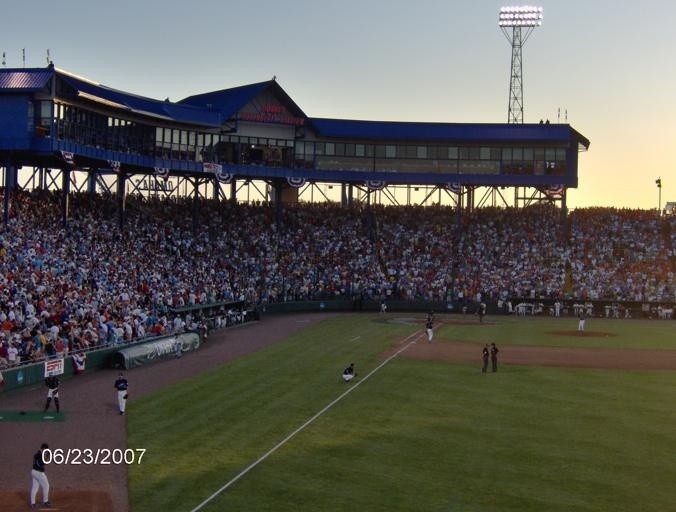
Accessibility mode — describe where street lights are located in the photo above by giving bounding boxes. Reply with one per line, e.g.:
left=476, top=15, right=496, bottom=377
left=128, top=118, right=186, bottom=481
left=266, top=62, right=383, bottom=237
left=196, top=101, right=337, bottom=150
left=497, top=5, right=544, bottom=124
left=656, top=176, right=661, bottom=211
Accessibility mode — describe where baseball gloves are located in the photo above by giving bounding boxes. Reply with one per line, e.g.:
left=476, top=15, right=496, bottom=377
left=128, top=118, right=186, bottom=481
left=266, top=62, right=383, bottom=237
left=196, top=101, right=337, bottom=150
left=53, top=389, right=58, bottom=394
left=123, top=394, right=128, bottom=399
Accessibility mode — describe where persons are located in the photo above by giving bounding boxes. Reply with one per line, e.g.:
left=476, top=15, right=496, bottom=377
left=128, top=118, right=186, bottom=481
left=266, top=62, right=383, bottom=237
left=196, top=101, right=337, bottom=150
left=269, top=200, right=676, bottom=303
left=577, top=311, right=586, bottom=335
left=342, top=364, right=356, bottom=382
left=44, top=372, right=62, bottom=413
left=497, top=301, right=672, bottom=319
left=480, top=301, right=486, bottom=315
left=489, top=343, right=499, bottom=372
left=1, top=181, right=269, bottom=372
left=481, top=344, right=489, bottom=373
left=30, top=443, right=52, bottom=509
left=426, top=319, right=434, bottom=343
left=114, top=372, right=129, bottom=415
left=478, top=305, right=484, bottom=322
left=381, top=303, right=387, bottom=313
